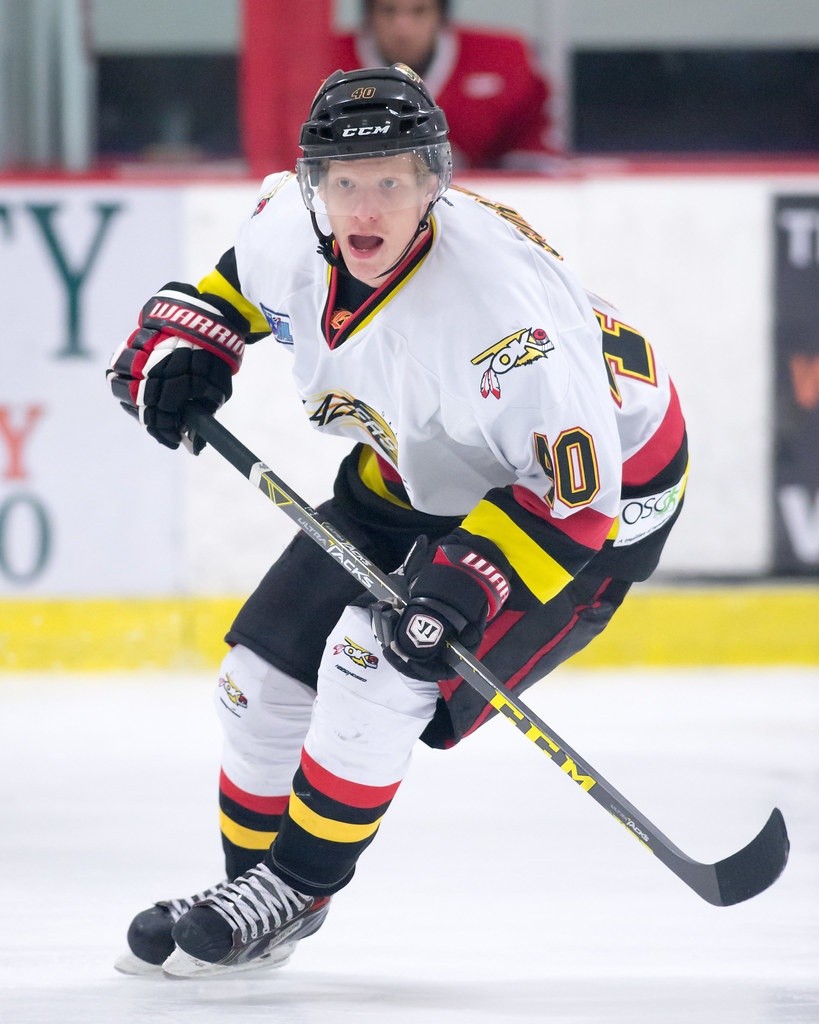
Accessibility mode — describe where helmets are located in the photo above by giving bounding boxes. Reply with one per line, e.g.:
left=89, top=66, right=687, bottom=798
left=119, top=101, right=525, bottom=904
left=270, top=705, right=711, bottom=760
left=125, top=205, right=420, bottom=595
left=294, top=62, right=449, bottom=185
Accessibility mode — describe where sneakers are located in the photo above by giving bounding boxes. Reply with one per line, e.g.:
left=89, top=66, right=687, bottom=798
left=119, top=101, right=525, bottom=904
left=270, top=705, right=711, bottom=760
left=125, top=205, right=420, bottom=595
left=114, top=878, right=231, bottom=975
left=161, top=861, right=332, bottom=978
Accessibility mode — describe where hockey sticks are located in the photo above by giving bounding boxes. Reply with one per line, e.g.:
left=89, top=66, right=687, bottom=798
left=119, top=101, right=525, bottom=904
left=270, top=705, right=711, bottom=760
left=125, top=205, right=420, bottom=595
left=184, top=405, right=791, bottom=908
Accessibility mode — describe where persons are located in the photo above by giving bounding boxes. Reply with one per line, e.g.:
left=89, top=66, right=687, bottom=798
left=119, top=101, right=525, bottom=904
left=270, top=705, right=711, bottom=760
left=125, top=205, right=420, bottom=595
left=331, top=1, right=558, bottom=171
left=111, top=63, right=688, bottom=981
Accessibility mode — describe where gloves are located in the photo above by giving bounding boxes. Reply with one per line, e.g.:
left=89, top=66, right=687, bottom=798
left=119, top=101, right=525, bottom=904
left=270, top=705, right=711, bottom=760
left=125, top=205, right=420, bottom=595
left=370, top=527, right=514, bottom=682
left=104, top=281, right=251, bottom=450
